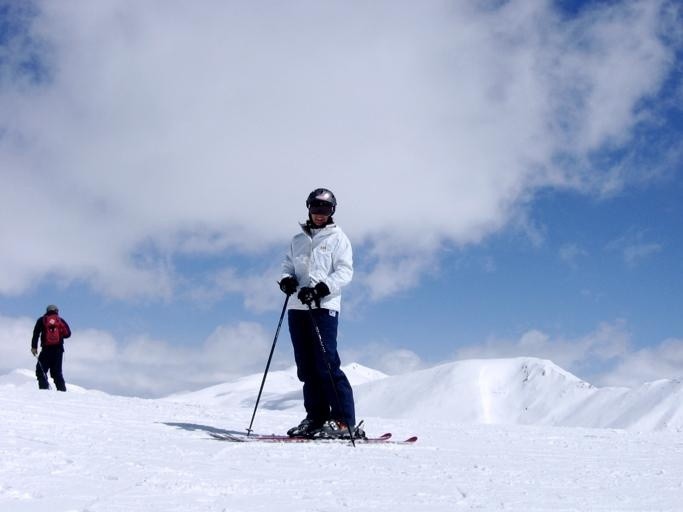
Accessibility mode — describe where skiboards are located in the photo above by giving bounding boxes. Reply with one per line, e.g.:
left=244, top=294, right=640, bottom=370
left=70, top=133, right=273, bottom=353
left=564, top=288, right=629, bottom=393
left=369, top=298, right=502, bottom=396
left=207, top=432, right=418, bottom=444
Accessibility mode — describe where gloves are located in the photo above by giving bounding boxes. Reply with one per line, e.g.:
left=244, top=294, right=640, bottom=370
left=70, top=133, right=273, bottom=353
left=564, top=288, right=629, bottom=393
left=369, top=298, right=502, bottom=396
left=280, top=277, right=297, bottom=295
left=298, top=284, right=324, bottom=304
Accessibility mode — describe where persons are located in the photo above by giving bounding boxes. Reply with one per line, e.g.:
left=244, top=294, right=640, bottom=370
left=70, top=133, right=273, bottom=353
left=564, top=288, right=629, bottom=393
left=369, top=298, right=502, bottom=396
left=277, top=188, right=365, bottom=439
left=30, top=303, right=72, bottom=393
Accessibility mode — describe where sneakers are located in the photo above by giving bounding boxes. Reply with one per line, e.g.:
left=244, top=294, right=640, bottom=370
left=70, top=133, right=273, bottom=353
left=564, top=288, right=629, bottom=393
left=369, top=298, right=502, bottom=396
left=288, top=417, right=321, bottom=437
left=313, top=421, right=366, bottom=439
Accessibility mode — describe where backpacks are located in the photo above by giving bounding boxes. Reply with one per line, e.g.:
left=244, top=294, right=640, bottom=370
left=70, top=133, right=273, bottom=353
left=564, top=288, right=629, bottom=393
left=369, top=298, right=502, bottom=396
left=43, top=316, right=63, bottom=346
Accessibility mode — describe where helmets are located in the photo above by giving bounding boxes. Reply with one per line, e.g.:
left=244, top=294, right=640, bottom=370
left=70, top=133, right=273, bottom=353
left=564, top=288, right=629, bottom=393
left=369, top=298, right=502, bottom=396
left=307, top=188, right=337, bottom=208
left=46, top=304, right=58, bottom=312
